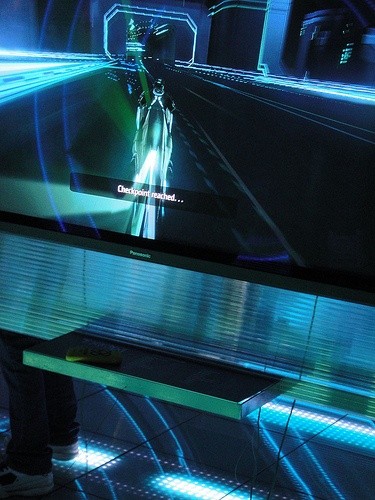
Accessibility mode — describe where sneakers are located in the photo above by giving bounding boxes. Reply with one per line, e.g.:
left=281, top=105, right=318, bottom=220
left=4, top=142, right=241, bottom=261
left=0, top=465, right=54, bottom=498
left=48, top=440, right=79, bottom=461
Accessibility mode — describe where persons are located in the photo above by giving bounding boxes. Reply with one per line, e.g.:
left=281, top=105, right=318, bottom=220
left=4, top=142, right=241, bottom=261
left=0, top=329, right=81, bottom=499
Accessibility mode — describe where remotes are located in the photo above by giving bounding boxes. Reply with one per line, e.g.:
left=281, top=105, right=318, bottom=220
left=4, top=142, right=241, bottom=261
left=65, top=346, right=123, bottom=364
